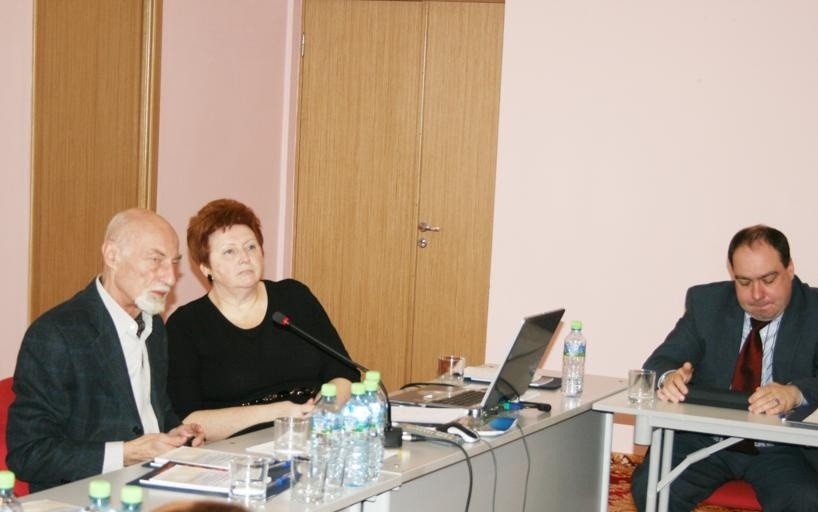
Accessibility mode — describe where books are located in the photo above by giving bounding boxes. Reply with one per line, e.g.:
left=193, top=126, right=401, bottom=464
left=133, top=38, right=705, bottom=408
left=132, top=441, right=281, bottom=496
left=462, top=361, right=545, bottom=382
left=469, top=375, right=555, bottom=387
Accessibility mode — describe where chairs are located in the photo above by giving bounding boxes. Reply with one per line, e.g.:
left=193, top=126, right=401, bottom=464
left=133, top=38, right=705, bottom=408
left=0, top=377, right=28, bottom=496
left=700, top=480, right=761, bottom=512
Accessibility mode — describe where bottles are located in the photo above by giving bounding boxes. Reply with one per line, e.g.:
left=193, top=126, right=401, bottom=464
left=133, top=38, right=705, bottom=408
left=84, top=479, right=117, bottom=512
left=119, top=484, right=145, bottom=511
left=562, top=319, right=587, bottom=396
left=314, top=369, right=388, bottom=493
left=0, top=469, right=23, bottom=512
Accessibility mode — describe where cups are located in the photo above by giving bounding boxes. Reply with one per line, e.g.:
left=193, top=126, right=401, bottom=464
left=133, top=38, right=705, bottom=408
left=626, top=368, right=657, bottom=406
left=290, top=454, right=327, bottom=503
left=274, top=416, right=312, bottom=454
left=437, top=353, right=466, bottom=387
left=228, top=452, right=270, bottom=511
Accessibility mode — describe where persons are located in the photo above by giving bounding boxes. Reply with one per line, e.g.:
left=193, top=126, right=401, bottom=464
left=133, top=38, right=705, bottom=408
left=628, top=224, right=818, bottom=511
left=162, top=198, right=362, bottom=444
left=4, top=207, right=208, bottom=493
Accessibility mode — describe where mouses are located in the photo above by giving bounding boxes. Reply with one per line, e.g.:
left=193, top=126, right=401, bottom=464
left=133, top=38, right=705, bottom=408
left=442, top=422, right=480, bottom=443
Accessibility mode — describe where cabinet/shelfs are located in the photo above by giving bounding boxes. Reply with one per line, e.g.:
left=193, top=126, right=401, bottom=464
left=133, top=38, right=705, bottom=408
left=291, top=0, right=506, bottom=394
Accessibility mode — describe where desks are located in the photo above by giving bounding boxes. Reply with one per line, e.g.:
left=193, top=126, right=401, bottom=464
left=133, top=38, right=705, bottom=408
left=0, top=368, right=630, bottom=512
left=593, top=382, right=818, bottom=512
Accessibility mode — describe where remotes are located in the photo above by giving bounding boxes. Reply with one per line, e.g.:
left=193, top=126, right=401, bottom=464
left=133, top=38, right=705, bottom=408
left=398, top=421, right=463, bottom=444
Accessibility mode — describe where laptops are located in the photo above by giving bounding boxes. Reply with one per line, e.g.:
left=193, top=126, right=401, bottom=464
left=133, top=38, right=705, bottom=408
left=388, top=308, right=565, bottom=420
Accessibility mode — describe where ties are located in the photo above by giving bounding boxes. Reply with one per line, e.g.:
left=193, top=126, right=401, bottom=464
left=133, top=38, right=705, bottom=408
left=724, top=317, right=772, bottom=454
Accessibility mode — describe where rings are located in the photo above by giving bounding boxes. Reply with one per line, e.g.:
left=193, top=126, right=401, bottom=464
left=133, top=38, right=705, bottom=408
left=773, top=397, right=780, bottom=406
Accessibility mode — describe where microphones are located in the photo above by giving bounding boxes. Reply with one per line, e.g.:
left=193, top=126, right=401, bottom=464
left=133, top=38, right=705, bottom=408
left=272, top=311, right=402, bottom=449
